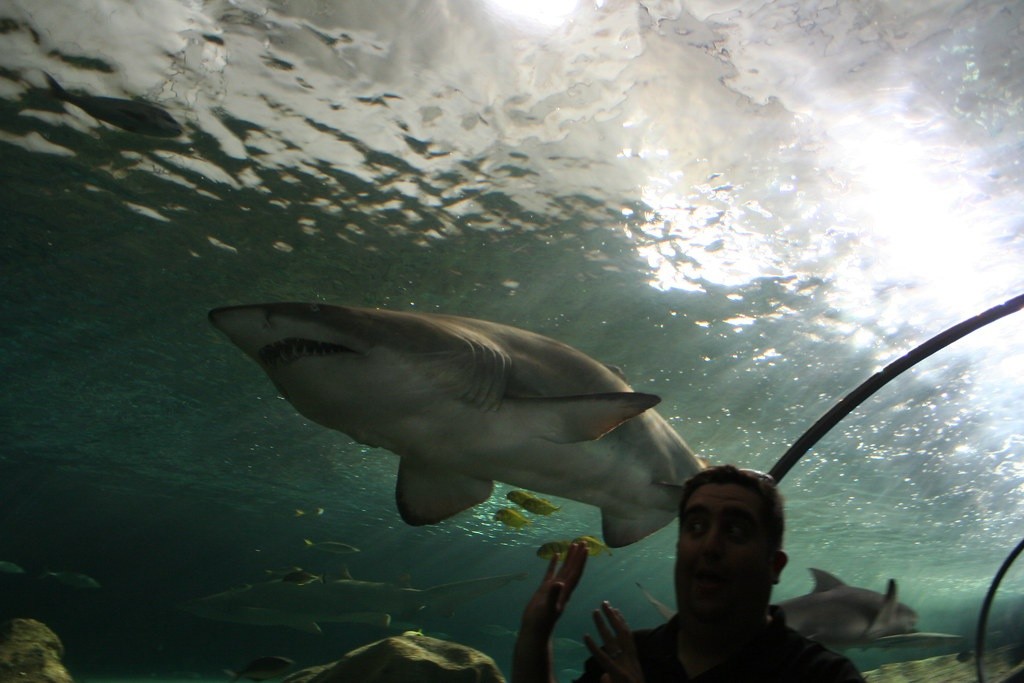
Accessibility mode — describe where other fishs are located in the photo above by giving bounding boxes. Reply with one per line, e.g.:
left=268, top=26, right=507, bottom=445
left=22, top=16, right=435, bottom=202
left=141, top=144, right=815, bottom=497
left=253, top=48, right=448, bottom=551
left=490, top=488, right=562, bottom=529
left=535, top=535, right=613, bottom=564
left=272, top=506, right=430, bottom=639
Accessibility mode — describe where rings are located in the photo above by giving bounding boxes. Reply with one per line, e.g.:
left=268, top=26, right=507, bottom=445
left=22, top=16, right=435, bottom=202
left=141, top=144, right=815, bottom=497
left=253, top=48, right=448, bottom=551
left=611, top=649, right=624, bottom=659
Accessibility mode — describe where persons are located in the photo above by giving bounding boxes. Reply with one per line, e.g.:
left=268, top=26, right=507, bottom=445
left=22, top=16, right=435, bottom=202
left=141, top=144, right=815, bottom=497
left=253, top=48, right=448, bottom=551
left=512, top=466, right=865, bottom=683
